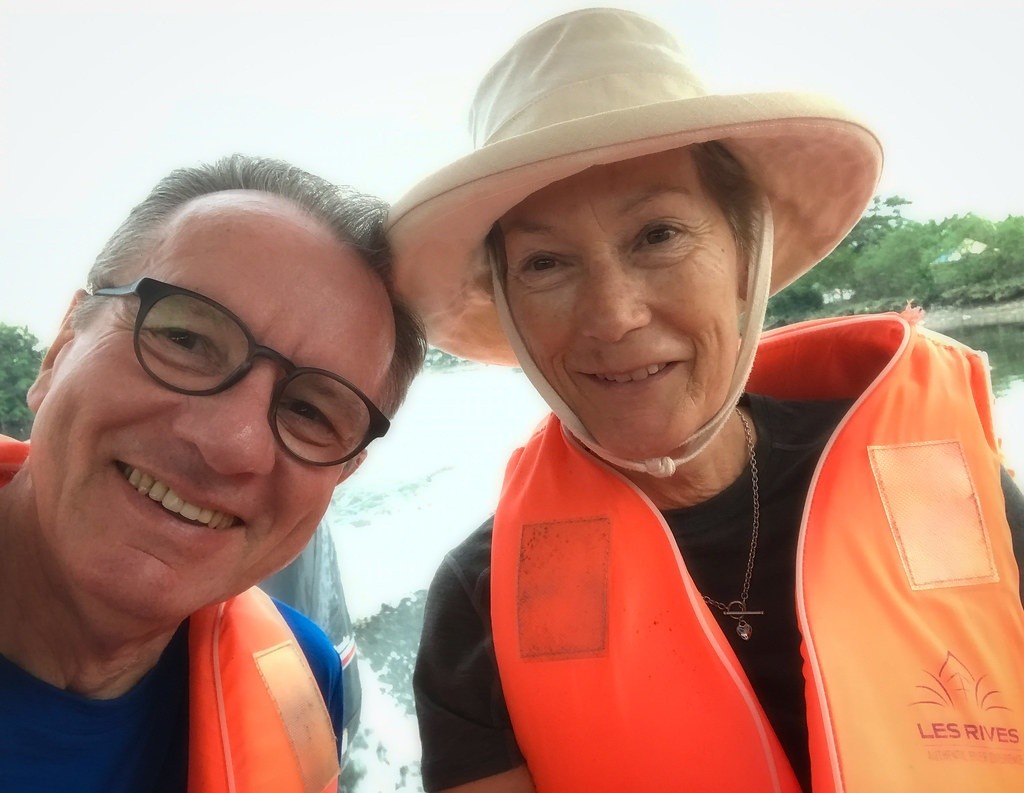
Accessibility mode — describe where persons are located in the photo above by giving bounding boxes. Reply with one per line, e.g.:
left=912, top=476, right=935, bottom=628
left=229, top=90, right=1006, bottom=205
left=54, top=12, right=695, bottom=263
left=0, top=158, right=426, bottom=793
left=387, top=7, right=1024, bottom=793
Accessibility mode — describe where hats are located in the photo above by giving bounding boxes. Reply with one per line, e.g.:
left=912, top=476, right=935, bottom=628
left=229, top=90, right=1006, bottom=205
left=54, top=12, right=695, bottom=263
left=385, top=8, right=885, bottom=366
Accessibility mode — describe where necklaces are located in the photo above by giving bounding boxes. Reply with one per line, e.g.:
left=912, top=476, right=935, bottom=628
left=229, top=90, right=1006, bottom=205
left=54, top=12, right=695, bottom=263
left=702, top=406, right=759, bottom=640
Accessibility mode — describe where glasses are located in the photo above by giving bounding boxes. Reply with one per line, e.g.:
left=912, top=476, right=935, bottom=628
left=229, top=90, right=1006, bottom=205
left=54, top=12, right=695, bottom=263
left=93, top=276, right=392, bottom=468
